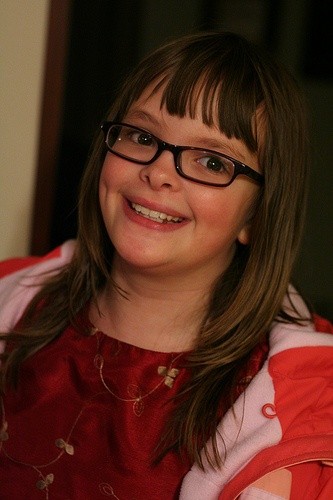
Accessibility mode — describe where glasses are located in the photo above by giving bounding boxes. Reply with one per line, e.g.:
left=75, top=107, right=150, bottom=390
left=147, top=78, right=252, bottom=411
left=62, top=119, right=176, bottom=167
left=99, top=120, right=263, bottom=190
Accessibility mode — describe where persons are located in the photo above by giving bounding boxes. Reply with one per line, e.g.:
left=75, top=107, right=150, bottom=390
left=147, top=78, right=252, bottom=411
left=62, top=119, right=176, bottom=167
left=0, top=29, right=333, bottom=500
